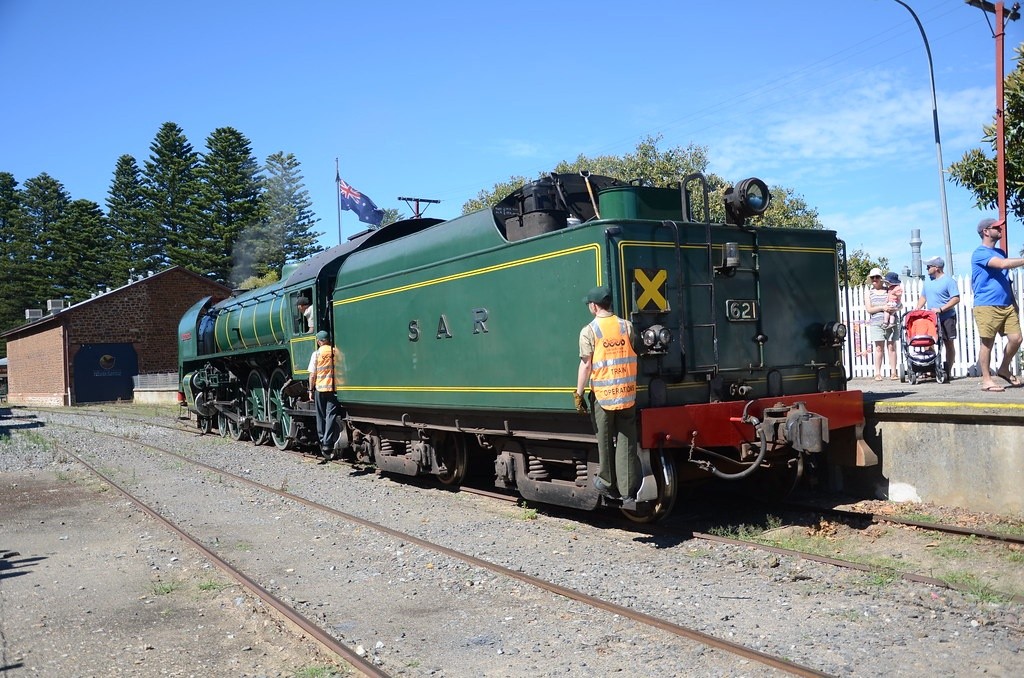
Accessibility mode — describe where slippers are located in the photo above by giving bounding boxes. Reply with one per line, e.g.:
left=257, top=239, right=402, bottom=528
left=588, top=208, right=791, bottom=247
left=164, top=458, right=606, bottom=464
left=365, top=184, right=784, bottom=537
left=997, top=373, right=1021, bottom=386
left=981, top=386, right=1005, bottom=392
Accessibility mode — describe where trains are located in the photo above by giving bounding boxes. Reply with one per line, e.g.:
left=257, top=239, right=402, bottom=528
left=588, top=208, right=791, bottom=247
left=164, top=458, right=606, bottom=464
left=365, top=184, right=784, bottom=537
left=176, top=169, right=878, bottom=528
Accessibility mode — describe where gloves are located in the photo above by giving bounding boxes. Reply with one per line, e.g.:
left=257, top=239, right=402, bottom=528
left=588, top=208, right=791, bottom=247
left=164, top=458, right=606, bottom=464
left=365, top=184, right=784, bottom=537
left=574, top=389, right=588, bottom=415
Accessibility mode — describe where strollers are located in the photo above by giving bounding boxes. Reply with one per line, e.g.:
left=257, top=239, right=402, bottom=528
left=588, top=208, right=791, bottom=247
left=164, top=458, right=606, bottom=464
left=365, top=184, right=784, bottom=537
left=898, top=309, right=949, bottom=385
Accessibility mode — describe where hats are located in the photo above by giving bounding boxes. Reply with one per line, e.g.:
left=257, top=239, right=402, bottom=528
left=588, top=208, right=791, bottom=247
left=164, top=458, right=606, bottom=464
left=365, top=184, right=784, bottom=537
left=294, top=296, right=309, bottom=306
left=977, top=218, right=1005, bottom=234
left=867, top=268, right=882, bottom=277
left=584, top=286, right=610, bottom=305
left=316, top=330, right=328, bottom=340
left=923, top=256, right=944, bottom=267
left=881, top=272, right=901, bottom=284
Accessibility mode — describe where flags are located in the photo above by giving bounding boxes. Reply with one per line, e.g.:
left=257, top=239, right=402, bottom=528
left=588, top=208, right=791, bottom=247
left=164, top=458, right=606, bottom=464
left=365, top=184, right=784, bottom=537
left=340, top=179, right=384, bottom=227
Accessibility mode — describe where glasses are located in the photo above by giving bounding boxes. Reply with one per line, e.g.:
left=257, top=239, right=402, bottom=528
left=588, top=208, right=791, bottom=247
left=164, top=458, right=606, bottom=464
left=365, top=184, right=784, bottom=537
left=870, top=275, right=880, bottom=279
left=927, top=265, right=935, bottom=268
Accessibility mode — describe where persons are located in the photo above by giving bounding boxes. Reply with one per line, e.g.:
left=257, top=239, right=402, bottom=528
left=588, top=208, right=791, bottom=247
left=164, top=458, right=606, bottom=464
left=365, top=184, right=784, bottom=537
left=574, top=286, right=637, bottom=504
left=915, top=256, right=960, bottom=383
left=971, top=218, right=1024, bottom=392
left=880, top=272, right=902, bottom=329
left=865, top=268, right=903, bottom=381
left=307, top=331, right=346, bottom=461
left=294, top=296, right=313, bottom=334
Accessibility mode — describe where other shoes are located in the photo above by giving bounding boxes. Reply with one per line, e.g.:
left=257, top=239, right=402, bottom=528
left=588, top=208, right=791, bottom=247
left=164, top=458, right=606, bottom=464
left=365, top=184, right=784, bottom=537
left=612, top=497, right=635, bottom=505
left=881, top=323, right=896, bottom=330
left=592, top=477, right=614, bottom=492
left=322, top=449, right=332, bottom=460
left=890, top=375, right=897, bottom=380
left=874, top=375, right=882, bottom=381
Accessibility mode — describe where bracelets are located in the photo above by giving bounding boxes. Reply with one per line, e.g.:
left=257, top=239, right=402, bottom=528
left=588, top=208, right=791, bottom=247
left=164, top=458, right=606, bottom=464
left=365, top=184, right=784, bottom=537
left=940, top=308, right=943, bottom=313
left=308, top=390, right=313, bottom=392
